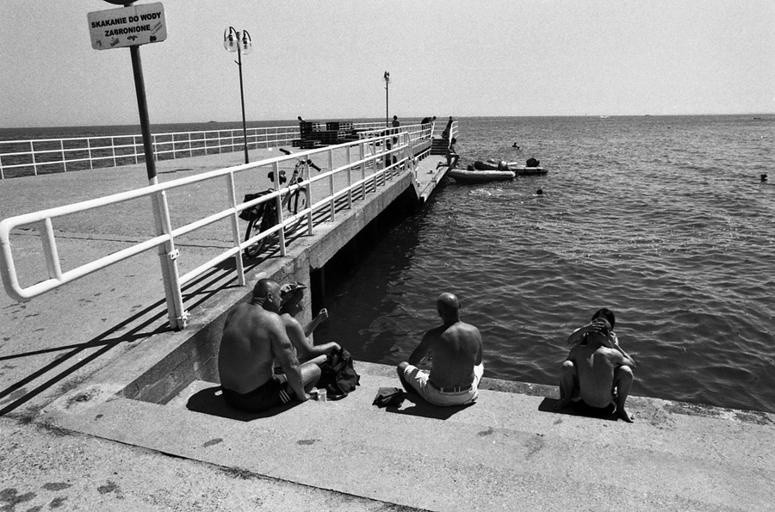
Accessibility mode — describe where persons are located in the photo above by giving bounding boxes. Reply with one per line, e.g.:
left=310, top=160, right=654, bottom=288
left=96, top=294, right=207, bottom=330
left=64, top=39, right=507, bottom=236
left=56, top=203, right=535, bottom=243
left=553, top=307, right=635, bottom=424
left=420, top=117, right=432, bottom=140
left=512, top=142, right=518, bottom=148
left=392, top=115, right=400, bottom=144
left=396, top=291, right=485, bottom=407
left=441, top=117, right=453, bottom=137
left=428, top=116, right=436, bottom=137
left=567, top=319, right=636, bottom=412
left=217, top=278, right=322, bottom=413
left=273, top=281, right=344, bottom=374
left=436, top=138, right=459, bottom=169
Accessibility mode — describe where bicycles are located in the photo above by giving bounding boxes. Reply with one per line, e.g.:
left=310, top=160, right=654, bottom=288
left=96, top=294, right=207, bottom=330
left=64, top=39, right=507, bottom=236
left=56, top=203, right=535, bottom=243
left=243, top=146, right=322, bottom=261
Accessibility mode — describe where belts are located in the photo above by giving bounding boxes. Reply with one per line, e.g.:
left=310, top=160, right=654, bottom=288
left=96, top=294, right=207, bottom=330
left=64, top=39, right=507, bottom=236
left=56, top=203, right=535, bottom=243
left=429, top=381, right=470, bottom=392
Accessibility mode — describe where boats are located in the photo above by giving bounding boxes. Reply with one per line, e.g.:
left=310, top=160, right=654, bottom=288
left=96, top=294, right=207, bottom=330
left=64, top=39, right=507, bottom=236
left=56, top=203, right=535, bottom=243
left=472, top=155, right=550, bottom=176
left=447, top=160, right=516, bottom=185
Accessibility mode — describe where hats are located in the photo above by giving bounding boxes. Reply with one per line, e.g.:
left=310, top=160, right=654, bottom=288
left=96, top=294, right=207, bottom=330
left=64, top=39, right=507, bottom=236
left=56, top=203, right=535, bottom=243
left=278, top=282, right=306, bottom=296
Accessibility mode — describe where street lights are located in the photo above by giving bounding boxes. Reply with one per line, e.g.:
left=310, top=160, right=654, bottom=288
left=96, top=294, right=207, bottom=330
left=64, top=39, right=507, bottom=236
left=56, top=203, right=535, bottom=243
left=382, top=68, right=392, bottom=130
left=223, top=23, right=255, bottom=163
